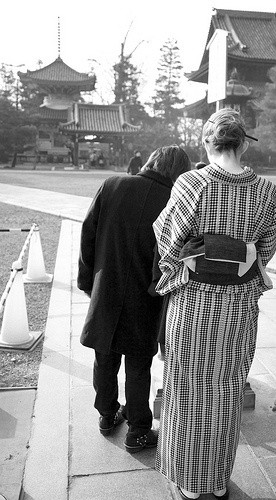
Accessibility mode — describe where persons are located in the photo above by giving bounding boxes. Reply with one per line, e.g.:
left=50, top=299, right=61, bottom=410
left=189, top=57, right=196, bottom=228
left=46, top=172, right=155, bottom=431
left=77, top=144, right=192, bottom=453
left=89, top=151, right=105, bottom=167
left=127, top=151, right=143, bottom=175
left=152, top=107, right=276, bottom=500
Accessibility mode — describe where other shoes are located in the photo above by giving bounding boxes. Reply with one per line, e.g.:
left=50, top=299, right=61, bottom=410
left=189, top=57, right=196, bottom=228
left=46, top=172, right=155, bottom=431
left=158, top=353, right=165, bottom=361
left=123, top=429, right=160, bottom=453
left=176, top=485, right=200, bottom=500
left=213, top=487, right=229, bottom=500
left=98, top=405, right=126, bottom=436
